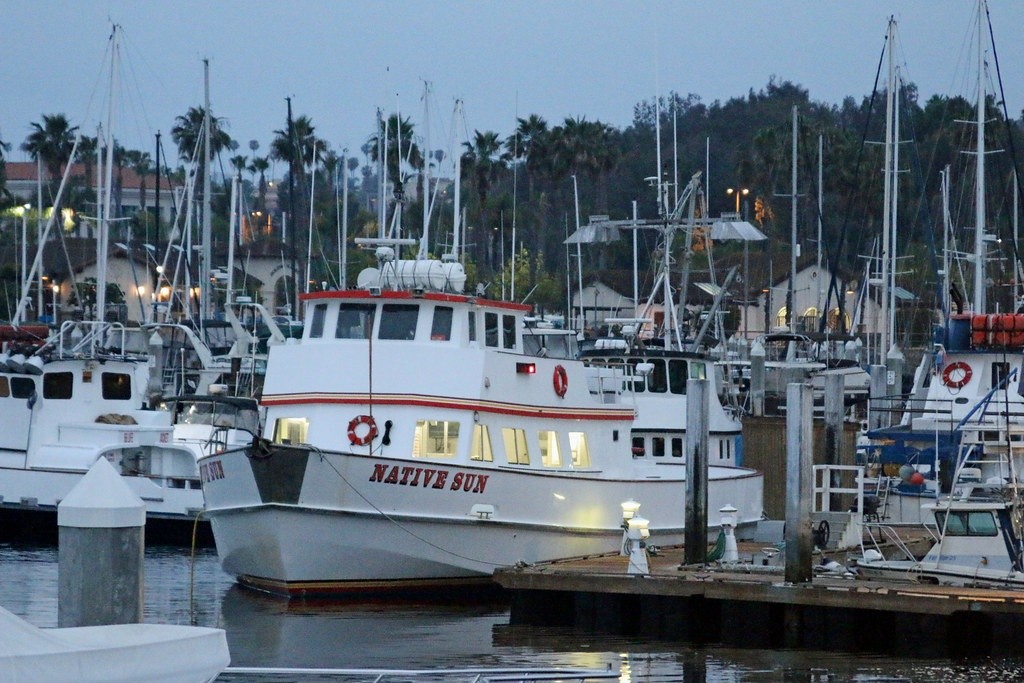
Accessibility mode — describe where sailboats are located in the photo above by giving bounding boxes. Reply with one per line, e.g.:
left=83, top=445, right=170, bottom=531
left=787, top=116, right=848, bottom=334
left=0, top=24, right=1023, bottom=589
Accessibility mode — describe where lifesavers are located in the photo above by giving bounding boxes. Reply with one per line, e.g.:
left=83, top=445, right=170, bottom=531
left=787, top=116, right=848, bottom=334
left=942, top=361, right=973, bottom=388
left=553, top=364, right=569, bottom=397
left=346, top=415, right=377, bottom=446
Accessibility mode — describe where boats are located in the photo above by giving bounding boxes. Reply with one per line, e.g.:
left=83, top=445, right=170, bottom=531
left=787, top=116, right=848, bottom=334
left=195, top=73, right=767, bottom=590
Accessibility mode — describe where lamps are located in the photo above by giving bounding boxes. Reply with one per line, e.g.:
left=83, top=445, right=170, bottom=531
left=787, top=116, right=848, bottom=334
left=621, top=498, right=641, bottom=556
left=624, top=516, right=651, bottom=574
left=0, top=352, right=45, bottom=375
